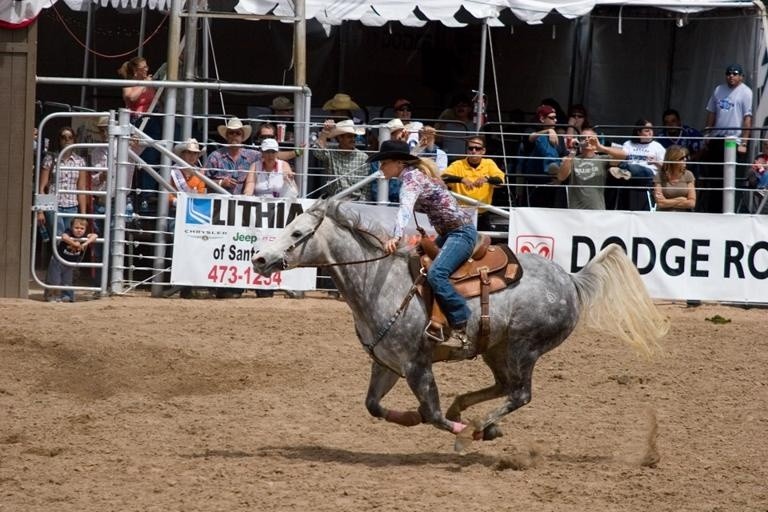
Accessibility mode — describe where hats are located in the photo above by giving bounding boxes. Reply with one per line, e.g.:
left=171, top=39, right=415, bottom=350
left=727, top=64, right=743, bottom=73
left=174, top=93, right=423, bottom=165
left=97, top=116, right=109, bottom=127
left=535, top=105, right=556, bottom=116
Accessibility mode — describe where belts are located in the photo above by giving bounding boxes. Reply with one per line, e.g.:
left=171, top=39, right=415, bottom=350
left=434, top=217, right=472, bottom=235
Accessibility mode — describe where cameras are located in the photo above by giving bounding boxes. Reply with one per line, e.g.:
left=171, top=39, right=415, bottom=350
left=572, top=141, right=589, bottom=149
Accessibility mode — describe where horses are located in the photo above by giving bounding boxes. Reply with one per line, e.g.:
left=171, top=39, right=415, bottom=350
left=251, top=193, right=671, bottom=453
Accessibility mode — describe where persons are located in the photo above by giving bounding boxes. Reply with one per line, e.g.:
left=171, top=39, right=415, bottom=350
left=169, top=93, right=697, bottom=211
left=364, top=140, right=478, bottom=348
left=750, top=153, right=768, bottom=215
left=117, top=58, right=157, bottom=208
left=699, top=63, right=753, bottom=213
left=32, top=115, right=146, bottom=300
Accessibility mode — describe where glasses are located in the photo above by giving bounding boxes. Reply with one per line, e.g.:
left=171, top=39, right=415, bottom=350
left=726, top=71, right=738, bottom=76
left=467, top=147, right=482, bottom=151
left=549, top=117, right=554, bottom=120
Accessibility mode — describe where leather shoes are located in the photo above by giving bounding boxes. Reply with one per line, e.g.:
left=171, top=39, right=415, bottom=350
left=428, top=322, right=467, bottom=341
